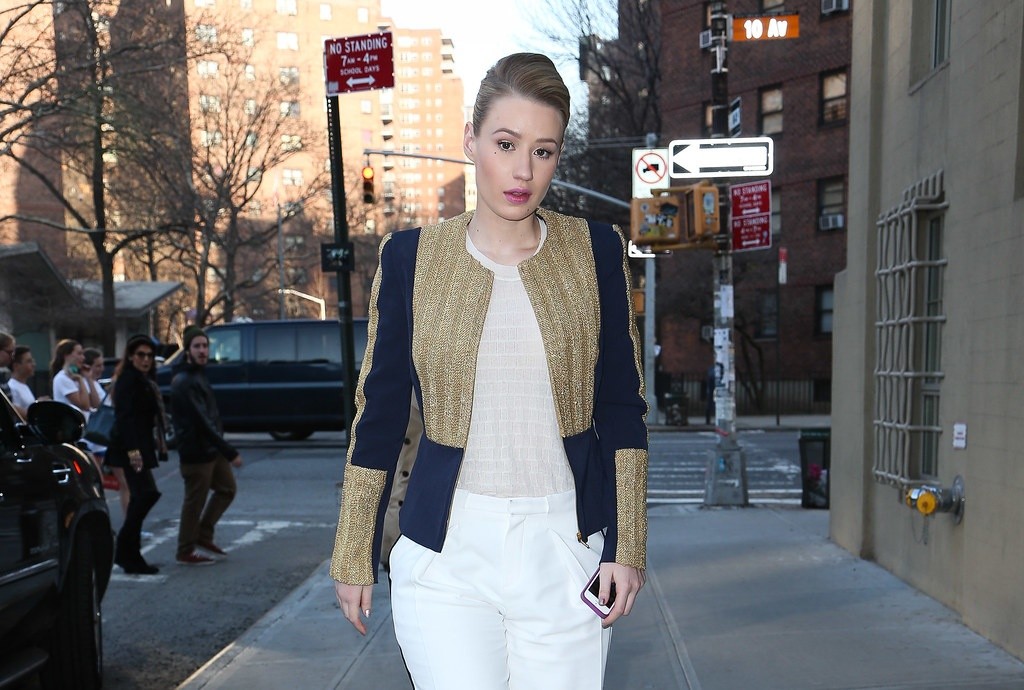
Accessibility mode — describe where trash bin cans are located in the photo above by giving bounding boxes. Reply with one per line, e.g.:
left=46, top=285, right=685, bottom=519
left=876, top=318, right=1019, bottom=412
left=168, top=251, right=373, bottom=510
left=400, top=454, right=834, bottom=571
left=663, top=393, right=688, bottom=427
left=798, top=427, right=830, bottom=509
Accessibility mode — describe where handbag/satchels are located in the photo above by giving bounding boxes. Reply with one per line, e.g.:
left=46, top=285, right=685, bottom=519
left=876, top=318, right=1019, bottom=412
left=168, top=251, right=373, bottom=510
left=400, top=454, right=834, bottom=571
left=84, top=386, right=116, bottom=447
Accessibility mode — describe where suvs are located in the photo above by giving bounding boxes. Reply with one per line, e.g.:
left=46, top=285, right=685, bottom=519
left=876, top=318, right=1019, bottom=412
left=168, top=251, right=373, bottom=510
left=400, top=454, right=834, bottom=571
left=154, top=314, right=371, bottom=450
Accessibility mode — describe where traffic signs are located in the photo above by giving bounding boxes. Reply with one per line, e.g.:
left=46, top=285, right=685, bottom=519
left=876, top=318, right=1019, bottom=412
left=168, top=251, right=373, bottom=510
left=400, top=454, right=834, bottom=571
left=325, top=31, right=395, bottom=96
left=730, top=213, right=773, bottom=254
left=730, top=179, right=774, bottom=220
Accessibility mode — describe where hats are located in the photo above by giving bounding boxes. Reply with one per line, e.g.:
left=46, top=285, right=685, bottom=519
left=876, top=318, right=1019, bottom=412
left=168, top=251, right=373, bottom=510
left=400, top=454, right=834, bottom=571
left=181, top=325, right=208, bottom=350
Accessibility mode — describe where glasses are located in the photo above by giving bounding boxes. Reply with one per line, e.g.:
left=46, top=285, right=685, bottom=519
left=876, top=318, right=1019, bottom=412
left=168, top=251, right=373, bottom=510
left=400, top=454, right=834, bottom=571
left=4, top=349, right=16, bottom=357
left=135, top=350, right=155, bottom=361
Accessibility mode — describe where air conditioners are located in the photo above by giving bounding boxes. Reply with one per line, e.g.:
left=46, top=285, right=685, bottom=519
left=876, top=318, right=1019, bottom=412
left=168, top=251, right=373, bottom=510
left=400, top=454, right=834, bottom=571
left=821, top=0, right=849, bottom=15
left=819, top=215, right=843, bottom=230
left=699, top=30, right=712, bottom=49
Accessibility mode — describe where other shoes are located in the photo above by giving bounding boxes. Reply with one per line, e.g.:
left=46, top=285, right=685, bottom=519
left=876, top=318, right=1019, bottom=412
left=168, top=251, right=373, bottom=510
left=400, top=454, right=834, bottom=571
left=195, top=540, right=228, bottom=557
left=175, top=552, right=214, bottom=565
left=112, top=545, right=159, bottom=575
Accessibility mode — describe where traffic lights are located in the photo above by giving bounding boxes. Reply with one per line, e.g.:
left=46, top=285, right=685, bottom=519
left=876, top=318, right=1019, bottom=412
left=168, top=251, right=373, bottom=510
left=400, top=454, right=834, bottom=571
left=683, top=184, right=720, bottom=242
left=361, top=167, right=376, bottom=204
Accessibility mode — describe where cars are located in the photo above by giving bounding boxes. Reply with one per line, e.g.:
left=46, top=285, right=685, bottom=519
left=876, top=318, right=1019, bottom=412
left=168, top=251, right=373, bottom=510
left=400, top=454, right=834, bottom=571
left=0, top=386, right=115, bottom=689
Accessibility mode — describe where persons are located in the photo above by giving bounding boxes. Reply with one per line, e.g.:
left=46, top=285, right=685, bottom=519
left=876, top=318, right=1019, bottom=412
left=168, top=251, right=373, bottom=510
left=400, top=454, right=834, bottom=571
left=50, top=339, right=153, bottom=540
left=0, top=330, right=27, bottom=419
left=8, top=345, right=51, bottom=410
left=169, top=328, right=241, bottom=566
left=329, top=53, right=650, bottom=690
left=103, top=337, right=170, bottom=575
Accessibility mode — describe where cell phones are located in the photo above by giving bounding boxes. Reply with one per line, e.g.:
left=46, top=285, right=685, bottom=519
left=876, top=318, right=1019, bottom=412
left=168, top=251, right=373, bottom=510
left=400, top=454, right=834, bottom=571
left=580, top=567, right=617, bottom=619
